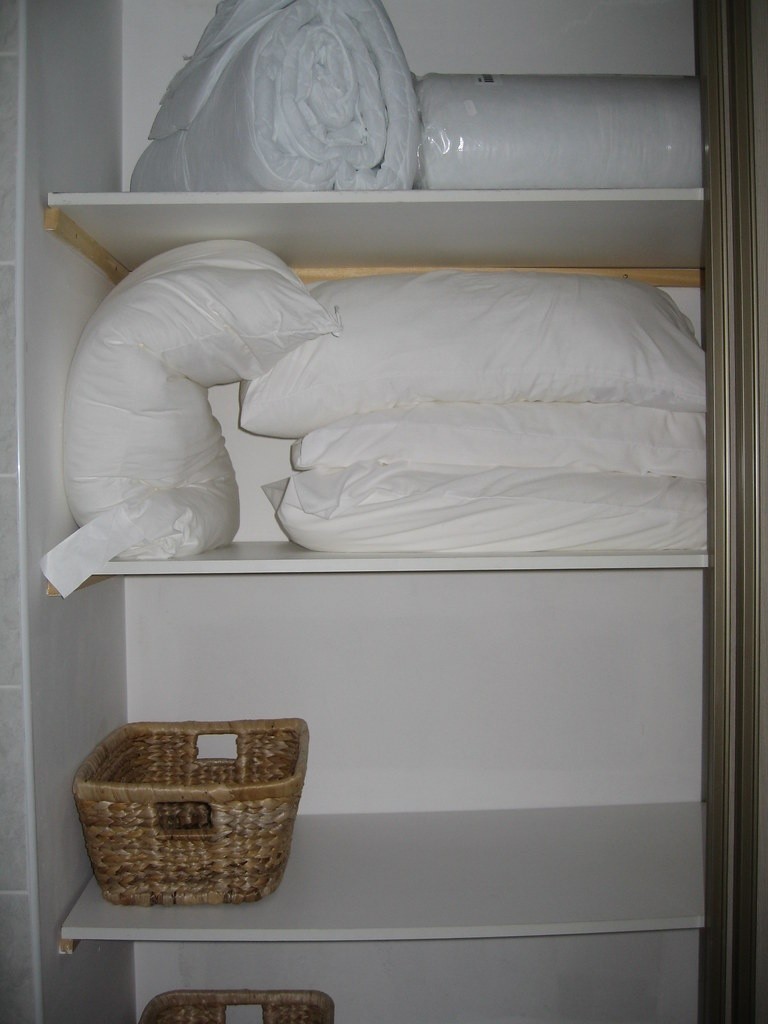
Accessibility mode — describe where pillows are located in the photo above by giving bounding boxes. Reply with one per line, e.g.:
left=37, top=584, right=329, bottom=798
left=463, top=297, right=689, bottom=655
left=58, top=237, right=708, bottom=563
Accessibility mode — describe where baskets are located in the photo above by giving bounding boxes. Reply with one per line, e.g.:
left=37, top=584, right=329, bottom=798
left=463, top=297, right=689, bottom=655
left=72, top=718, right=309, bottom=906
left=138, top=987, right=336, bottom=1023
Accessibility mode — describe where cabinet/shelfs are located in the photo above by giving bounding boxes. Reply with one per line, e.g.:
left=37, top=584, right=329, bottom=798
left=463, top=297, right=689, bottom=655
left=12, top=1, right=724, bottom=1024
